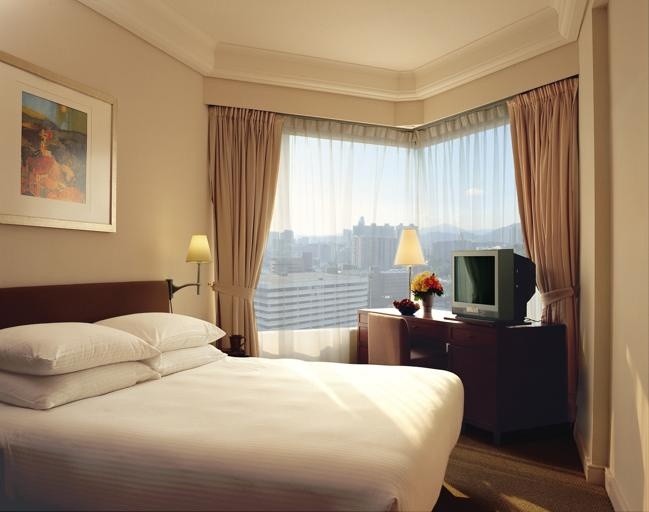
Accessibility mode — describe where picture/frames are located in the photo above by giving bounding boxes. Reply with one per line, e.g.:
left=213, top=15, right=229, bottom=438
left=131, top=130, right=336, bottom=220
left=0, top=48, right=117, bottom=232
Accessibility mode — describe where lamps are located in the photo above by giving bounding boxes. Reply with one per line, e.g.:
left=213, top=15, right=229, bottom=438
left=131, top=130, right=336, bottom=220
left=167, top=236, right=213, bottom=299
left=393, top=230, right=427, bottom=296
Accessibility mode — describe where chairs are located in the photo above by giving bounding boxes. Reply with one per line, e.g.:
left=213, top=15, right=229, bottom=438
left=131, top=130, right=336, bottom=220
left=368, top=313, right=434, bottom=368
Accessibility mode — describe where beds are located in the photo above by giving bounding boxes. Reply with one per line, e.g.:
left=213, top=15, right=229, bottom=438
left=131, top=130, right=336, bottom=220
left=0, top=280, right=465, bottom=512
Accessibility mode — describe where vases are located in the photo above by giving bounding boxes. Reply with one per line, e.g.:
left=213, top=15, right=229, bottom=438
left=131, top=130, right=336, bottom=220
left=421, top=294, right=435, bottom=312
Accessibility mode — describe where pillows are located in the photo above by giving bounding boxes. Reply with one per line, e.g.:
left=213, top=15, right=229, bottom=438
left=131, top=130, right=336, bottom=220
left=0, top=313, right=230, bottom=409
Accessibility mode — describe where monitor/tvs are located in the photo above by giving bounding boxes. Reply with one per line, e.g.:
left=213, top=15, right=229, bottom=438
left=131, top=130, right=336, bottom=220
left=451, top=248, right=536, bottom=324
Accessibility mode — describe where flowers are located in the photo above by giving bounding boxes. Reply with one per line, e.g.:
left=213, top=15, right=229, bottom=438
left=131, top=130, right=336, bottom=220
left=411, top=271, right=445, bottom=301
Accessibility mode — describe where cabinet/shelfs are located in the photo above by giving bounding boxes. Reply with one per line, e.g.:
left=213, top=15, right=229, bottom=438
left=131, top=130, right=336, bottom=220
left=358, top=307, right=566, bottom=448
